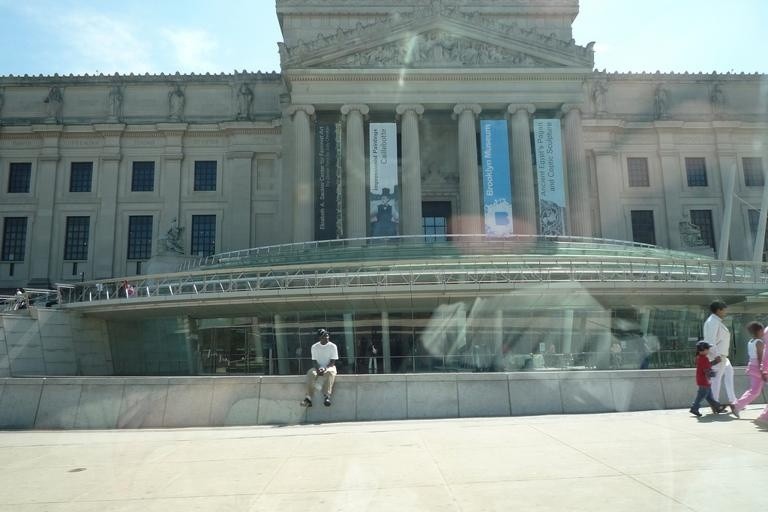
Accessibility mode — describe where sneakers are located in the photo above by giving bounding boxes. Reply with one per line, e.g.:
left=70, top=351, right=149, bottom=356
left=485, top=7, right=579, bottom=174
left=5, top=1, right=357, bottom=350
left=731, top=405, right=739, bottom=417
left=323, top=394, right=331, bottom=406
left=690, top=408, right=702, bottom=416
left=300, top=398, right=312, bottom=407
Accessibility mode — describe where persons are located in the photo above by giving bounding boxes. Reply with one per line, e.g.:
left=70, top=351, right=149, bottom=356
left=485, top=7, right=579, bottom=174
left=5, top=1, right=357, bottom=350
left=15, top=288, right=21, bottom=295
left=728, top=321, right=765, bottom=417
left=608, top=327, right=659, bottom=370
left=300, top=328, right=339, bottom=407
left=295, top=341, right=306, bottom=374
left=756, top=327, right=768, bottom=422
left=703, top=300, right=746, bottom=412
left=370, top=194, right=398, bottom=236
left=689, top=340, right=726, bottom=417
left=123, top=279, right=130, bottom=288
left=368, top=342, right=378, bottom=368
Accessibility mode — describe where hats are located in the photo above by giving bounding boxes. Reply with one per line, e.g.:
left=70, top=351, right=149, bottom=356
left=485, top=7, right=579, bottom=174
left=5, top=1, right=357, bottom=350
left=318, top=328, right=330, bottom=338
left=696, top=341, right=712, bottom=348
